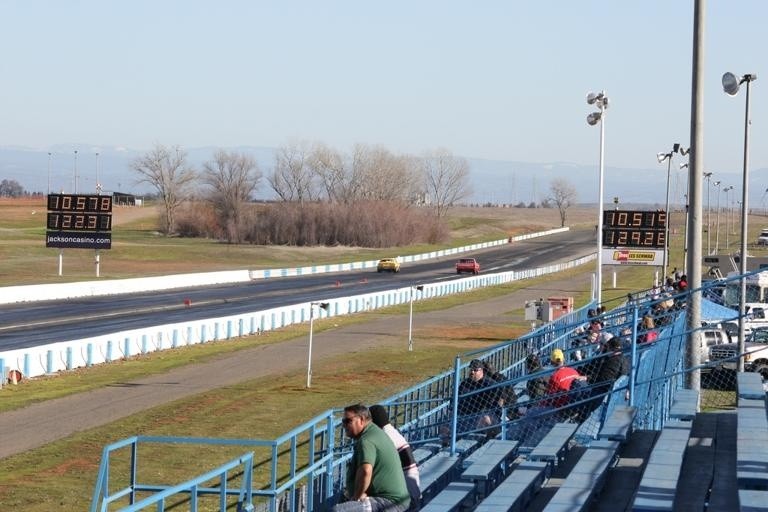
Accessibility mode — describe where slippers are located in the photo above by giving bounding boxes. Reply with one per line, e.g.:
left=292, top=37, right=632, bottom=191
left=341, top=415, right=357, bottom=424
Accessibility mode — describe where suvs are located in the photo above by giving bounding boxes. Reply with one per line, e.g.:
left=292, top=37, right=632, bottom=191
left=697, top=269, right=767, bottom=382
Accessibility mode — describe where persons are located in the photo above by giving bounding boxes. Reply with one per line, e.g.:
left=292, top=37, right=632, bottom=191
left=331, top=404, right=411, bottom=512
left=369, top=406, right=424, bottom=510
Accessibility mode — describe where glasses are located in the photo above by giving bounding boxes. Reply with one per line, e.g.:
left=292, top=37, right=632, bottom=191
left=470, top=368, right=480, bottom=371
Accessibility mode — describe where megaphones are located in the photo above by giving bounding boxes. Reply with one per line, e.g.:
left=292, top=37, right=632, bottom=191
left=657, top=139, right=736, bottom=194
left=720, top=70, right=748, bottom=95
left=586, top=90, right=608, bottom=126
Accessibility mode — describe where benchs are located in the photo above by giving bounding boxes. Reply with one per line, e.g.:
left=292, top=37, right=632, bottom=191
left=412, top=282, right=767, bottom=512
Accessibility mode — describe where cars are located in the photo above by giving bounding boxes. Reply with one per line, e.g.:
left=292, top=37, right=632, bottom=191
left=377, top=257, right=400, bottom=272
left=456, top=258, right=480, bottom=275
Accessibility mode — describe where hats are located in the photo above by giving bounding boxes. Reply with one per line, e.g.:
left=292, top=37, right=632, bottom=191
left=550, top=348, right=564, bottom=363
left=468, top=359, right=485, bottom=368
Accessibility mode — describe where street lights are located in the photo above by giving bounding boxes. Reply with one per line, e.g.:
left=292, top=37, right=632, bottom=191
left=724, top=70, right=756, bottom=372
left=655, top=142, right=742, bottom=299
left=586, top=92, right=609, bottom=312
left=307, top=302, right=329, bottom=386
left=408, top=283, right=423, bottom=351
